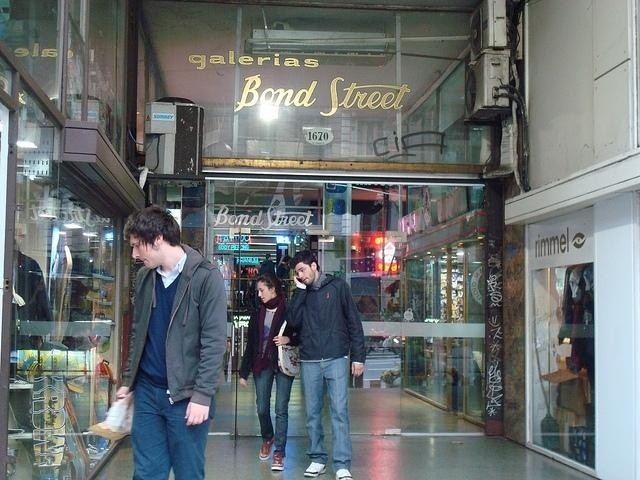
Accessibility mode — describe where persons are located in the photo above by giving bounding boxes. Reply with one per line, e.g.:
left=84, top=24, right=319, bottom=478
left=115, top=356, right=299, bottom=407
left=237, top=251, right=291, bottom=309
left=236, top=269, right=302, bottom=472
left=113, top=205, right=230, bottom=480
left=283, top=247, right=368, bottom=479
left=3, top=240, right=56, bottom=480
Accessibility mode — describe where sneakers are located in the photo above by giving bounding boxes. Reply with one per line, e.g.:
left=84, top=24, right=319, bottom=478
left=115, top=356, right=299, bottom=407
left=336, top=469, right=353, bottom=480
left=257, top=433, right=274, bottom=462
left=304, top=460, right=327, bottom=477
left=270, top=449, right=286, bottom=472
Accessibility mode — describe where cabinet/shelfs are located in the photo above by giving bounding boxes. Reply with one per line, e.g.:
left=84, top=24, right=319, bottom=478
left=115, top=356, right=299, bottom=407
left=363, top=348, right=401, bottom=389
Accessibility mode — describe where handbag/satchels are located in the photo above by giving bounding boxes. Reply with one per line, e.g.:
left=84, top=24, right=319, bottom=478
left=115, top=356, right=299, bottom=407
left=274, top=340, right=303, bottom=377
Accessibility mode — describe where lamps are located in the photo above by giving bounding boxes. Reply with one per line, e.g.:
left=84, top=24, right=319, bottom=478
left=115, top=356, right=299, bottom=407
left=243, top=29, right=389, bottom=67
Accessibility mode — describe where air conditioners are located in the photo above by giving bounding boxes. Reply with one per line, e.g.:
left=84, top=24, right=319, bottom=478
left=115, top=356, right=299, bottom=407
left=146, top=95, right=204, bottom=188
left=466, top=0, right=513, bottom=125
